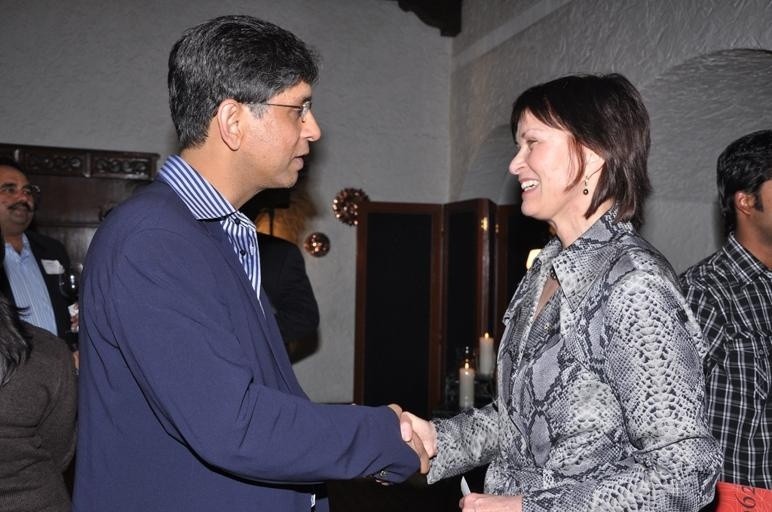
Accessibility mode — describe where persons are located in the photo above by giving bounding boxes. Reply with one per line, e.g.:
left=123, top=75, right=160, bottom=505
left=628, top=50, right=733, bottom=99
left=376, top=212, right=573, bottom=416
left=0, top=294, right=78, bottom=511
left=234, top=230, right=320, bottom=367
left=71, top=15, right=430, bottom=511
left=673, top=126, right=772, bottom=511
left=375, top=72, right=724, bottom=511
left=1, top=161, right=84, bottom=356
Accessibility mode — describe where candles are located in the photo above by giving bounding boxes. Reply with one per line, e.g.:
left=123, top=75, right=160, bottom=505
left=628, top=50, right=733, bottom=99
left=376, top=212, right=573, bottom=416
left=479, top=338, right=494, bottom=374
left=459, top=367, right=475, bottom=408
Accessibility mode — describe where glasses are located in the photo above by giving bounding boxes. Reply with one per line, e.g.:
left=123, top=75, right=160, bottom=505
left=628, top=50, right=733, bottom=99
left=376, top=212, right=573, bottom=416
left=243, top=99, right=313, bottom=122
